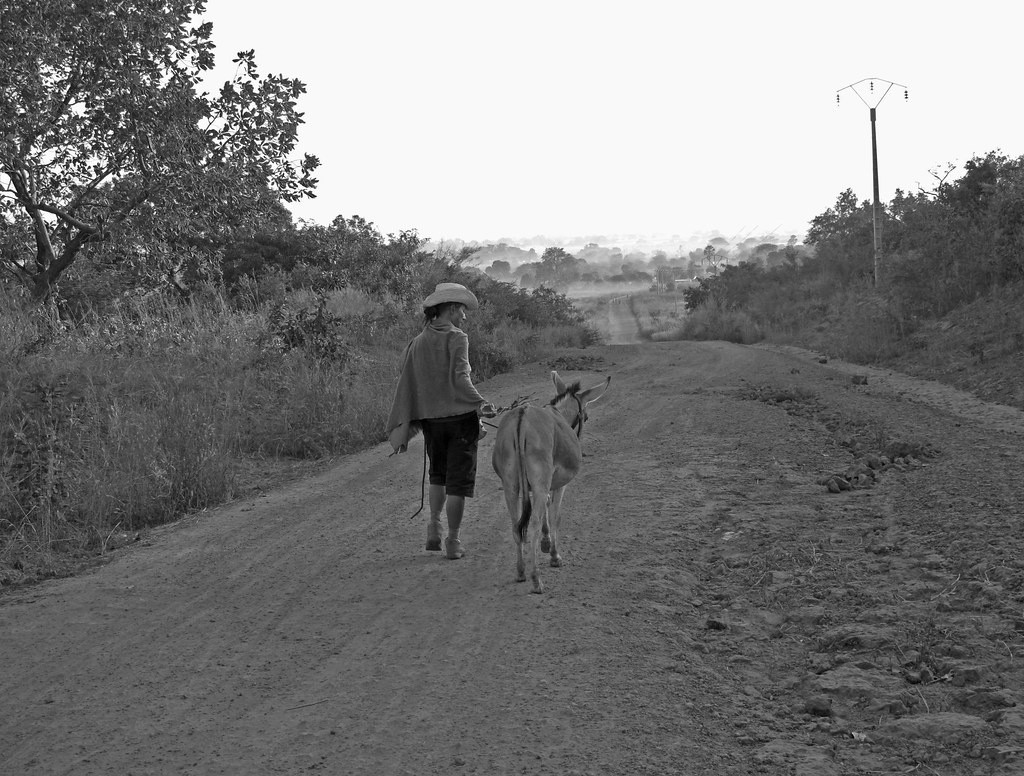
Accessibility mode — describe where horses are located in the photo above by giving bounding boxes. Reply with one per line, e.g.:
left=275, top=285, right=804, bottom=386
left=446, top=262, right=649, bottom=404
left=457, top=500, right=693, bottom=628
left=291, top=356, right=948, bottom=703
left=492, top=370, right=611, bottom=594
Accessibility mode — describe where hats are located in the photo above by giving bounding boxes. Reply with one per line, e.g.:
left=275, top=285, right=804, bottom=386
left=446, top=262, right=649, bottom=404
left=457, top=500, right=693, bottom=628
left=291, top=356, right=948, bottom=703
left=421, top=282, right=479, bottom=311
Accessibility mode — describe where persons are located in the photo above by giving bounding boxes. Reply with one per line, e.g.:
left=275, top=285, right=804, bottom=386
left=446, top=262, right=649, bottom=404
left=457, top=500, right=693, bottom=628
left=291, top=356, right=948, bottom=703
left=385, top=283, right=496, bottom=560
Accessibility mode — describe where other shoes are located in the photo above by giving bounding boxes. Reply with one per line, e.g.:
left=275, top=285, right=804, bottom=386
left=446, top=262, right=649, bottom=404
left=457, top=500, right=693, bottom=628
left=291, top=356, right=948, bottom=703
left=444, top=537, right=466, bottom=559
left=425, top=522, right=443, bottom=550
